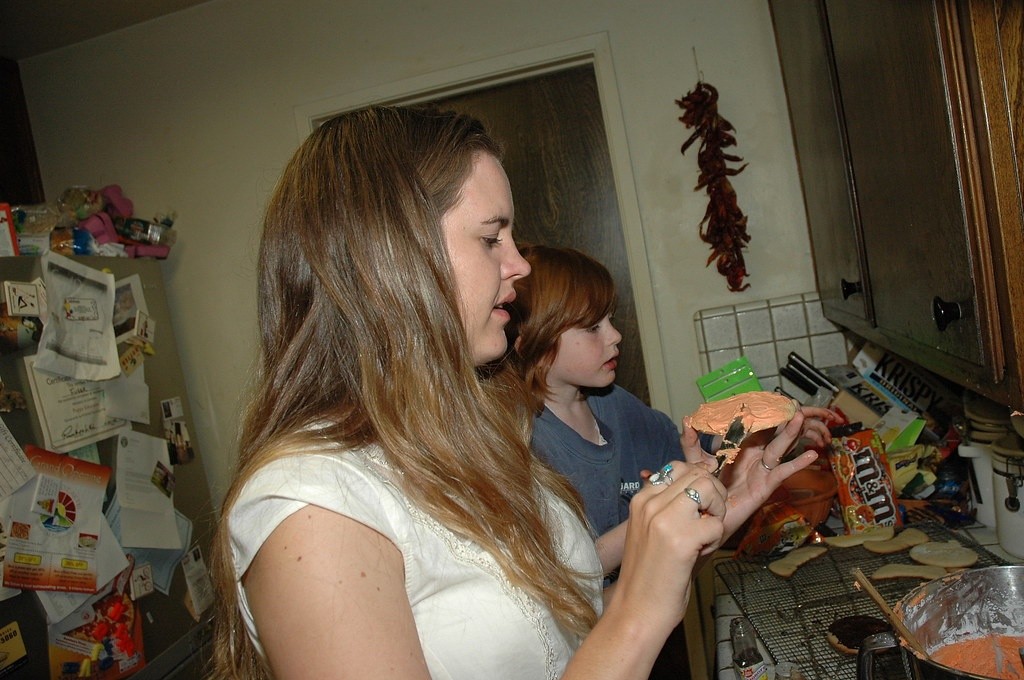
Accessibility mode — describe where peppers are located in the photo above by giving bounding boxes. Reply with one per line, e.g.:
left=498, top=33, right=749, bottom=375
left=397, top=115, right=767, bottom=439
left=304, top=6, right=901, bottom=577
left=674, top=81, right=753, bottom=292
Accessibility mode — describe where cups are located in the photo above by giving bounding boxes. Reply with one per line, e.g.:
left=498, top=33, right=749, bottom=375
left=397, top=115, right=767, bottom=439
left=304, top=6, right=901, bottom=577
left=991, top=436, right=1024, bottom=560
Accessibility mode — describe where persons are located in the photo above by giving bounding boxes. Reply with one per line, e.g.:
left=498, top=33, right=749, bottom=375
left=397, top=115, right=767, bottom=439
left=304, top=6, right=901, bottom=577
left=504, top=242, right=844, bottom=587
left=202, top=103, right=819, bottom=680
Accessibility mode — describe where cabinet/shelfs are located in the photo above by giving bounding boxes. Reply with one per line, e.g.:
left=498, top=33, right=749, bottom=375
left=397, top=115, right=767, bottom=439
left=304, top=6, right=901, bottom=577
left=767, top=0, right=1024, bottom=415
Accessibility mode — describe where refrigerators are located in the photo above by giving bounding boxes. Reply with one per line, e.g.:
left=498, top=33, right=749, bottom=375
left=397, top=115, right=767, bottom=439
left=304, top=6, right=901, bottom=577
left=0, top=255, right=217, bottom=680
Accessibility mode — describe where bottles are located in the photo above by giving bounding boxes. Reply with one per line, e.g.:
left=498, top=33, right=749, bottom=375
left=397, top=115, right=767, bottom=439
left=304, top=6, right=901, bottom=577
left=730, top=617, right=769, bottom=680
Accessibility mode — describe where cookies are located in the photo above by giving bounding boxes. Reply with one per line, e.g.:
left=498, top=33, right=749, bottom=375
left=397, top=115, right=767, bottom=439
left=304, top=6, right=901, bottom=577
left=827, top=631, right=890, bottom=655
left=770, top=524, right=979, bottom=579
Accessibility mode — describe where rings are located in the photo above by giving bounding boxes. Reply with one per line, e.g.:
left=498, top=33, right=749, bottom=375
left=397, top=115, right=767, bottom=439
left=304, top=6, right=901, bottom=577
left=648, top=464, right=674, bottom=487
left=762, top=458, right=771, bottom=470
left=684, top=487, right=702, bottom=504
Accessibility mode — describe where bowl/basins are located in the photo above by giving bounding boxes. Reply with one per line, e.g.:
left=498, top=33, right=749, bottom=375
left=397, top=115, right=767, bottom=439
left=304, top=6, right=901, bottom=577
left=717, top=470, right=838, bottom=550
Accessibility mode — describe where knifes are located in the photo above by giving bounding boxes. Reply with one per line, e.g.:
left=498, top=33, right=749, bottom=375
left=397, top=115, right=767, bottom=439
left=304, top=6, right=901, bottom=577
left=774, top=351, right=838, bottom=406
left=713, top=401, right=750, bottom=478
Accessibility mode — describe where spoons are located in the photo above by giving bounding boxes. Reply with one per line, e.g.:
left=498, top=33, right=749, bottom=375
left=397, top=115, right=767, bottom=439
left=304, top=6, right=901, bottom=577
left=953, top=416, right=983, bottom=504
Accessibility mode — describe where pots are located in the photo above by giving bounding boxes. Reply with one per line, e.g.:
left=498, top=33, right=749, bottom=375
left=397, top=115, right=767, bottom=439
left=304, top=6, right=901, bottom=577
left=855, top=566, right=1024, bottom=680
